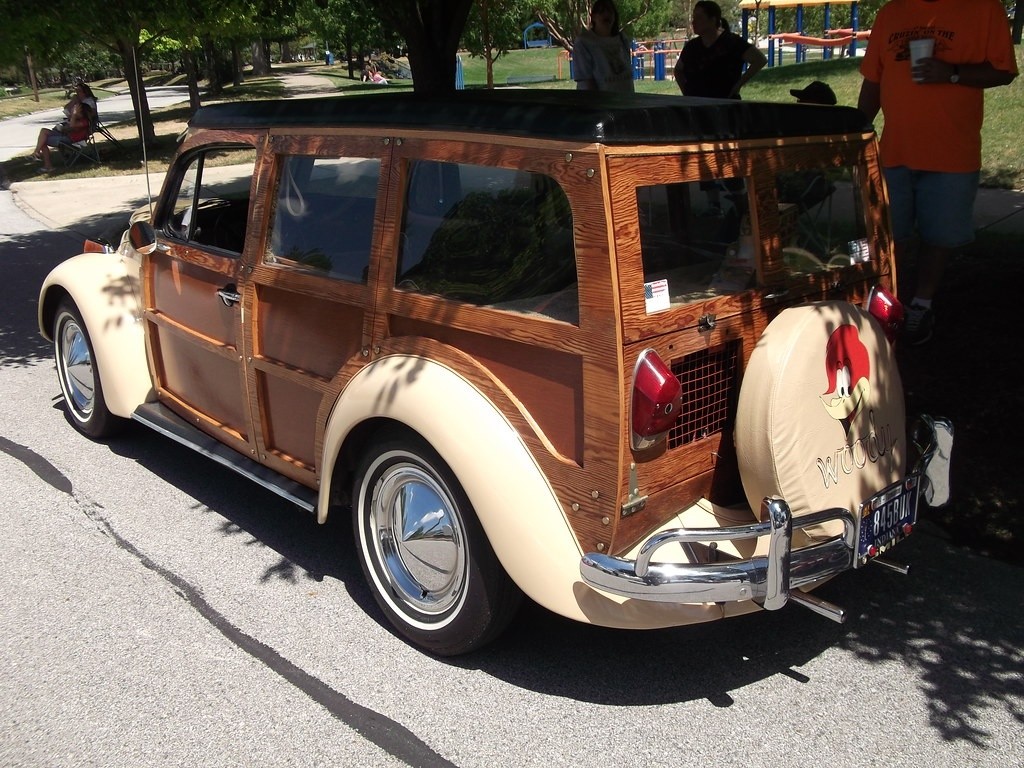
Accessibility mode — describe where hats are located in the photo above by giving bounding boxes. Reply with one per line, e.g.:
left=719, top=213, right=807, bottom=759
left=790, top=80, right=839, bottom=105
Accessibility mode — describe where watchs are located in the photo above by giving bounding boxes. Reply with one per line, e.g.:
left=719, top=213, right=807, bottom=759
left=949, top=63, right=960, bottom=85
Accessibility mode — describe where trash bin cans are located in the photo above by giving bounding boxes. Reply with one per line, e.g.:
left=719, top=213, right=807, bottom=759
left=329, top=54, right=335, bottom=64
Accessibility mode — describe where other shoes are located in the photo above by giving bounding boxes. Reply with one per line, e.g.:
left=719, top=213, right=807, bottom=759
left=24, top=153, right=43, bottom=166
left=32, top=167, right=57, bottom=176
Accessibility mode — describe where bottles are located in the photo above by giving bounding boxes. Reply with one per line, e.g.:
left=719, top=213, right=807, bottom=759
left=56, top=122, right=64, bottom=131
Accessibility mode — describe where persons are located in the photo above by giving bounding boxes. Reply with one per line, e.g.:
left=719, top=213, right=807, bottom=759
left=673, top=1, right=768, bottom=213
left=25, top=102, right=92, bottom=174
left=360, top=60, right=388, bottom=85
left=723, top=80, right=837, bottom=243
left=570, top=0, right=635, bottom=94
left=51, top=83, right=98, bottom=163
left=834, top=0, right=1019, bottom=346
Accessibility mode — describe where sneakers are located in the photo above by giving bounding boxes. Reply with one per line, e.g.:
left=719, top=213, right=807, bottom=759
left=903, top=304, right=936, bottom=346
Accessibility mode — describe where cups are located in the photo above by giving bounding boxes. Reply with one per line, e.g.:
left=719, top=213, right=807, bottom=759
left=909, top=38, right=935, bottom=81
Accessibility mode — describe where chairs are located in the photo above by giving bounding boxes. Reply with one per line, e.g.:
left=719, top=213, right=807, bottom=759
left=777, top=171, right=837, bottom=256
left=58, top=111, right=127, bottom=170
